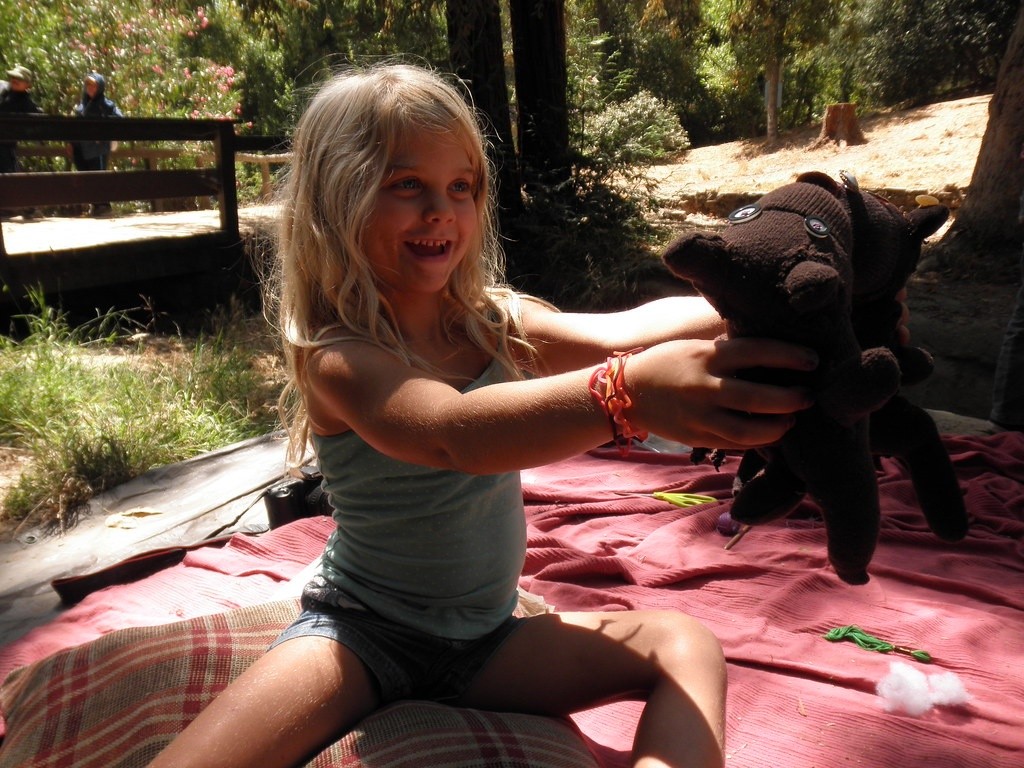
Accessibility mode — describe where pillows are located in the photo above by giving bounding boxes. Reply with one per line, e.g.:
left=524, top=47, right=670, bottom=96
left=0, top=598, right=603, bottom=768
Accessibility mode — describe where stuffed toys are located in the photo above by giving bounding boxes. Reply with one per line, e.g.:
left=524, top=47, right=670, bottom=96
left=660, top=171, right=971, bottom=586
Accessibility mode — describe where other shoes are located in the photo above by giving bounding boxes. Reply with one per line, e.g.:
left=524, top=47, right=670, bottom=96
left=84, top=203, right=112, bottom=218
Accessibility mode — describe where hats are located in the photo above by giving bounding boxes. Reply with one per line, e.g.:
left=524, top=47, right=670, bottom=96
left=6, top=66, right=32, bottom=83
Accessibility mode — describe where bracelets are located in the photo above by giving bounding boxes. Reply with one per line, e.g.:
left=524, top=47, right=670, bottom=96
left=589, top=346, right=651, bottom=449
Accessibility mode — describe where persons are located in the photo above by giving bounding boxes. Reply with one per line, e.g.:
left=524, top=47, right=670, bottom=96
left=0, top=66, right=44, bottom=216
left=143, top=61, right=807, bottom=768
left=66, top=73, right=123, bottom=216
